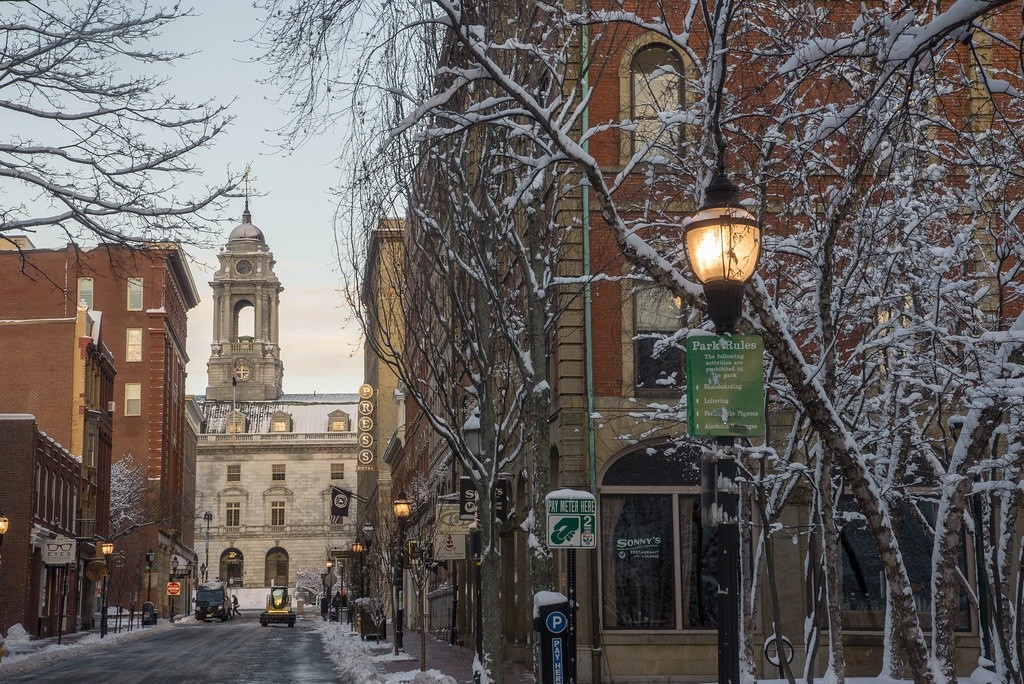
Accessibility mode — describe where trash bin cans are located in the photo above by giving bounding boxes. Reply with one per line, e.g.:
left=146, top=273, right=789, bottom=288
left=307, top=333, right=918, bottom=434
left=360, top=597, right=386, bottom=641
left=142, top=601, right=159, bottom=629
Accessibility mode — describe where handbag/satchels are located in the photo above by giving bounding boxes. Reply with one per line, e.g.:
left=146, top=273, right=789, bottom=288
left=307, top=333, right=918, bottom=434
left=235, top=603, right=240, bottom=608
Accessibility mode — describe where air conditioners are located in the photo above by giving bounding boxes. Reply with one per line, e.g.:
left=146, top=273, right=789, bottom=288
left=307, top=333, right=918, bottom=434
left=107, top=401, right=116, bottom=412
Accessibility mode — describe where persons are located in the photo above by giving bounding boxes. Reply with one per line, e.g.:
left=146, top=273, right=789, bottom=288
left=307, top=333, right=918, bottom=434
left=231, top=595, right=241, bottom=618
left=320, top=597, right=329, bottom=622
left=333, top=591, right=342, bottom=623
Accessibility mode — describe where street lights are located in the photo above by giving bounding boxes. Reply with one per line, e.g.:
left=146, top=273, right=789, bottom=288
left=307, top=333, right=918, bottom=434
left=170, top=556, right=179, bottom=623
left=101, top=538, right=114, bottom=635
left=682, top=173, right=768, bottom=684
left=146, top=551, right=155, bottom=601
left=394, top=491, right=412, bottom=654
left=325, top=559, right=333, bottom=605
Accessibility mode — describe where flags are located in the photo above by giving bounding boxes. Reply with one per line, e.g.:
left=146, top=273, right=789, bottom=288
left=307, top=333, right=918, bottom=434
left=329, top=487, right=352, bottom=525
left=232, top=361, right=237, bottom=386
left=245, top=164, right=251, bottom=179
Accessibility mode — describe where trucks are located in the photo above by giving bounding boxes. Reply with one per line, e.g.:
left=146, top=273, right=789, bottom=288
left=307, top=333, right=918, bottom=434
left=193, top=582, right=229, bottom=622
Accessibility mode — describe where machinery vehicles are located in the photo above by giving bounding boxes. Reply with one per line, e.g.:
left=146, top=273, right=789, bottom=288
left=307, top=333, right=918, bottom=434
left=259, top=586, right=296, bottom=628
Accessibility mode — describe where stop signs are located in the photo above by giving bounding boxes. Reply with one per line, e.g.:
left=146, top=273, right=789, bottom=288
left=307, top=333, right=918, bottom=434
left=167, top=582, right=181, bottom=595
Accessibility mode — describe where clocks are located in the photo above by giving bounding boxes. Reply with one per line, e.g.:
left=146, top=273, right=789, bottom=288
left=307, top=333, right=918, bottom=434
left=236, top=260, right=252, bottom=274
